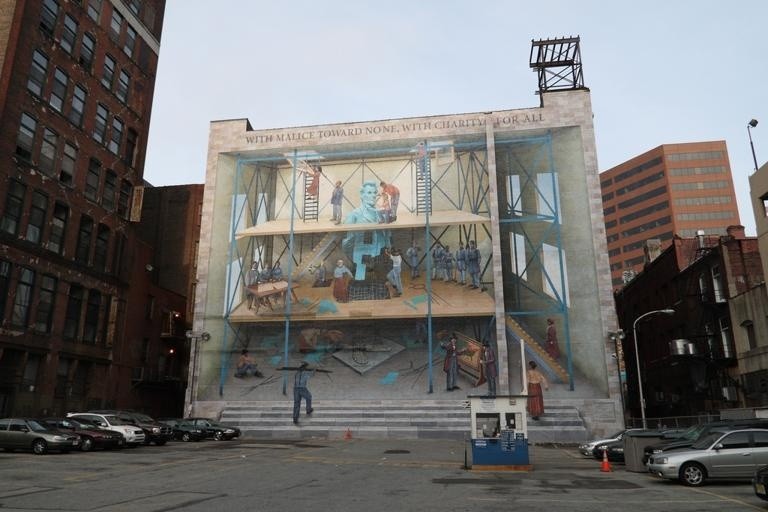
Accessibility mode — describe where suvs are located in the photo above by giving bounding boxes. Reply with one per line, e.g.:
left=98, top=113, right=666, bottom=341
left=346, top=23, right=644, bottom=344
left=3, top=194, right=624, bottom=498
left=89, top=409, right=173, bottom=447
left=61, top=411, right=146, bottom=449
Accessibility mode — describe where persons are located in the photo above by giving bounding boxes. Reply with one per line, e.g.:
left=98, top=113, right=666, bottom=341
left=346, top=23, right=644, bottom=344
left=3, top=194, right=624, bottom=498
left=246, top=261, right=260, bottom=310
left=307, top=166, right=322, bottom=202
left=383, top=247, right=403, bottom=302
left=292, top=361, right=317, bottom=422
left=268, top=343, right=283, bottom=370
left=406, top=240, right=482, bottom=289
left=478, top=341, right=498, bottom=397
left=334, top=258, right=353, bottom=303
left=416, top=142, right=429, bottom=179
left=525, top=360, right=549, bottom=419
left=258, top=262, right=273, bottom=306
left=438, top=332, right=466, bottom=392
left=342, top=181, right=392, bottom=281
left=273, top=260, right=283, bottom=281
left=237, top=348, right=262, bottom=378
left=330, top=180, right=343, bottom=226
left=380, top=181, right=400, bottom=224
left=544, top=318, right=561, bottom=359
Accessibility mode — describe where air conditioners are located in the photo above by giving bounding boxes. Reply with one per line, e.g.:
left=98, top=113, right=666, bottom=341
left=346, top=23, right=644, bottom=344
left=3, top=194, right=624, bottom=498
left=686, top=344, right=697, bottom=355
left=670, top=339, right=690, bottom=353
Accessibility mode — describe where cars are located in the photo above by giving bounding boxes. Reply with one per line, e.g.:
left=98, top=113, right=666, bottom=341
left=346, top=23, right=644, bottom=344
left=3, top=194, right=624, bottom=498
left=753, top=464, right=768, bottom=502
left=593, top=431, right=645, bottom=458
left=578, top=428, right=648, bottom=456
left=644, top=423, right=768, bottom=487
left=154, top=418, right=208, bottom=443
left=184, top=417, right=241, bottom=441
left=606, top=441, right=624, bottom=461
left=0, top=418, right=82, bottom=456
left=38, top=417, right=126, bottom=451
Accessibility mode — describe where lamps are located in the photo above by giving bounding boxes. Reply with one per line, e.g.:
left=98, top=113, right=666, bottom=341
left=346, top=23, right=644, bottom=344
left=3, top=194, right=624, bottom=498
left=143, top=262, right=151, bottom=271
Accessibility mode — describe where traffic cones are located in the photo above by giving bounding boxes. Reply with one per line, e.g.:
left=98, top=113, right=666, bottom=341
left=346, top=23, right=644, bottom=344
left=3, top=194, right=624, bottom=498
left=343, top=426, right=352, bottom=439
left=598, top=449, right=613, bottom=473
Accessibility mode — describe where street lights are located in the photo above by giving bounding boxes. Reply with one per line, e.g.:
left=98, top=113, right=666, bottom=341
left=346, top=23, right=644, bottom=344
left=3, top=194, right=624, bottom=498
left=608, top=328, right=629, bottom=431
left=186, top=330, right=211, bottom=418
left=633, top=308, right=677, bottom=426
left=746, top=118, right=759, bottom=171
